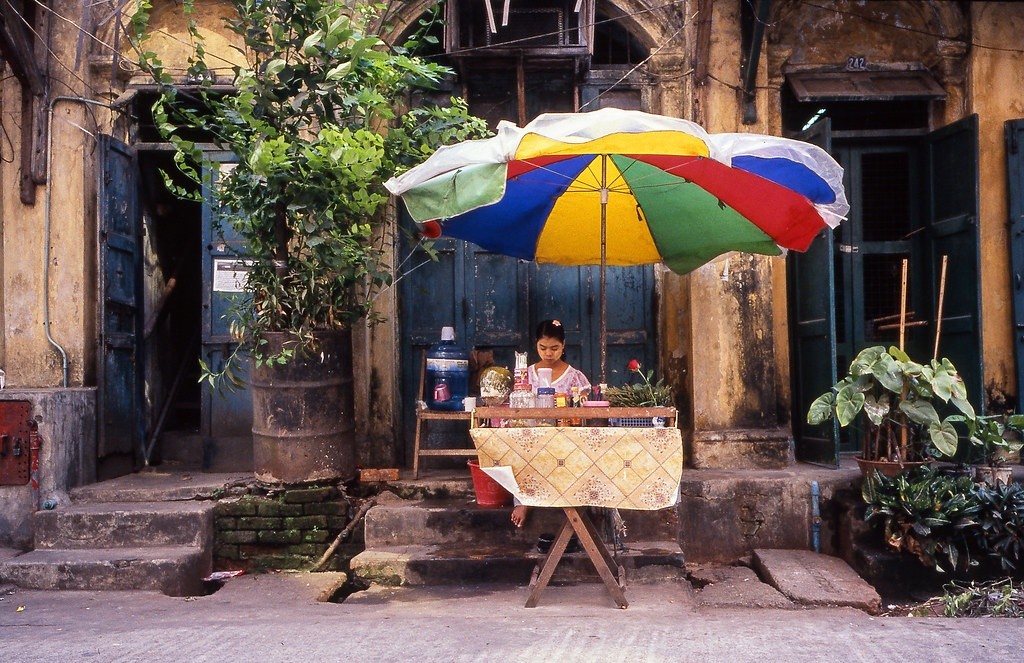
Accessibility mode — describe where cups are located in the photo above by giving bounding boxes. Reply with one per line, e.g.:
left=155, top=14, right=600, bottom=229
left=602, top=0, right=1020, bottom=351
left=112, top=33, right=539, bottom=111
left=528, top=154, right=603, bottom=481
left=462, top=397, right=476, bottom=412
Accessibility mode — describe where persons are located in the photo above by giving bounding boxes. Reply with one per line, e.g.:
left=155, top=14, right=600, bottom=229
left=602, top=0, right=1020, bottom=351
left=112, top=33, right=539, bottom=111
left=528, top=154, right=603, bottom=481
left=511, top=320, right=591, bottom=526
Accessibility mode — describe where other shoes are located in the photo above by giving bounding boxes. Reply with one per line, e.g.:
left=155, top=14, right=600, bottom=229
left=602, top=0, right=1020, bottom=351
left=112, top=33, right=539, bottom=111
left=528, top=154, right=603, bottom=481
left=537, top=533, right=578, bottom=552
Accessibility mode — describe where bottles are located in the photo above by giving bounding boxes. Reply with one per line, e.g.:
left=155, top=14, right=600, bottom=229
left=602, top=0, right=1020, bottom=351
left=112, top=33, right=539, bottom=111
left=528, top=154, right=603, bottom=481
left=424, top=326, right=470, bottom=412
left=569, top=386, right=583, bottom=427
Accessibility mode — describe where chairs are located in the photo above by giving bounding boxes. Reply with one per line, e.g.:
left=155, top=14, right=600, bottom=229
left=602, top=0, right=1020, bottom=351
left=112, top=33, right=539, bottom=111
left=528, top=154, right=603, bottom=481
left=412, top=350, right=494, bottom=481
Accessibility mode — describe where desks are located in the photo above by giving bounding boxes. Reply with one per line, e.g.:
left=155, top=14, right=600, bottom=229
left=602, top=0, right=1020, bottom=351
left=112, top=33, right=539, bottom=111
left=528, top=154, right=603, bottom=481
left=470, top=427, right=683, bottom=611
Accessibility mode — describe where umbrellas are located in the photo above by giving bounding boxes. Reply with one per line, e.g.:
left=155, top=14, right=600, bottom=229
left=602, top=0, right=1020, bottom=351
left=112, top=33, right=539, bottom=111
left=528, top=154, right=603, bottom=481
left=381, top=105, right=852, bottom=403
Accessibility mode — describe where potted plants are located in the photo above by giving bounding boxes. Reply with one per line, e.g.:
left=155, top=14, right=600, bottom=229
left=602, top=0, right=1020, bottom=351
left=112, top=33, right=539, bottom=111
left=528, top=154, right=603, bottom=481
left=806, top=345, right=978, bottom=484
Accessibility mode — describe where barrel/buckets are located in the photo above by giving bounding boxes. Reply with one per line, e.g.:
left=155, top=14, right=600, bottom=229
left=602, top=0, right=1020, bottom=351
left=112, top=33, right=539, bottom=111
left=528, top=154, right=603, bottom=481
left=466, top=458, right=514, bottom=507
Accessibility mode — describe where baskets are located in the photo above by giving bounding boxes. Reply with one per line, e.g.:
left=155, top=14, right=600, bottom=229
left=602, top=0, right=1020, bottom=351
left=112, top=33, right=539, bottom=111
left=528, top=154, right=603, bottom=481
left=608, top=417, right=666, bottom=428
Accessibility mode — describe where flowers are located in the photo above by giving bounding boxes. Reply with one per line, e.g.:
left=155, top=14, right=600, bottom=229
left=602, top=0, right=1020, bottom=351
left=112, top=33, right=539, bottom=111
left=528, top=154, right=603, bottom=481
left=626, top=358, right=658, bottom=406
